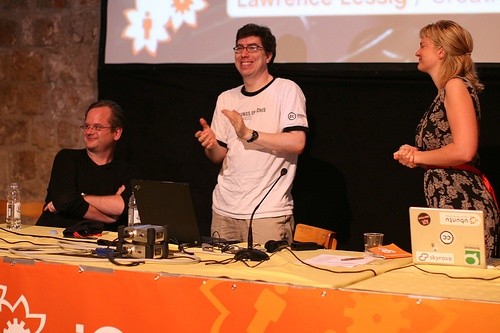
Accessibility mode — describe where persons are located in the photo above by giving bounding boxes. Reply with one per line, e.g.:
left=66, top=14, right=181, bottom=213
left=35, top=100, right=132, bottom=228
left=394, top=20, right=500, bottom=258
left=195, top=23, right=308, bottom=242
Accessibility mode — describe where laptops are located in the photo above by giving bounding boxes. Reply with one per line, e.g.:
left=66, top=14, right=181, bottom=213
left=408, top=206, right=500, bottom=268
left=131, top=177, right=241, bottom=247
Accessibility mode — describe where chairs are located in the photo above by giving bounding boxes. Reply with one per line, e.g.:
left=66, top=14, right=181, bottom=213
left=293, top=223, right=337, bottom=250
left=0, top=195, right=45, bottom=223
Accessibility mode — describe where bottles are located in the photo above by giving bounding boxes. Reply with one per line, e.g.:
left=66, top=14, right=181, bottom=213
left=127, top=191, right=141, bottom=226
left=6, top=183, right=22, bottom=231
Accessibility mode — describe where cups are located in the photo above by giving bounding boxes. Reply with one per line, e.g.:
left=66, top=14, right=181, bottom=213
left=364, top=233, right=384, bottom=265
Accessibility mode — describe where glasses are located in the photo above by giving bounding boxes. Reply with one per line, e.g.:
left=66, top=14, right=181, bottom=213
left=233, top=45, right=264, bottom=52
left=80, top=124, right=113, bottom=131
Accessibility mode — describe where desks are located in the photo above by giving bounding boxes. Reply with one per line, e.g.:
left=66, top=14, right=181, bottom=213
left=0, top=220, right=500, bottom=333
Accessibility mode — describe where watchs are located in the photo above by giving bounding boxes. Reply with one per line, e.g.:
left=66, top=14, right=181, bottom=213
left=247, top=130, right=259, bottom=143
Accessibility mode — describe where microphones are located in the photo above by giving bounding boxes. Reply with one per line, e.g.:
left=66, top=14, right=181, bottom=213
left=263, top=238, right=287, bottom=252
left=233, top=167, right=288, bottom=261
